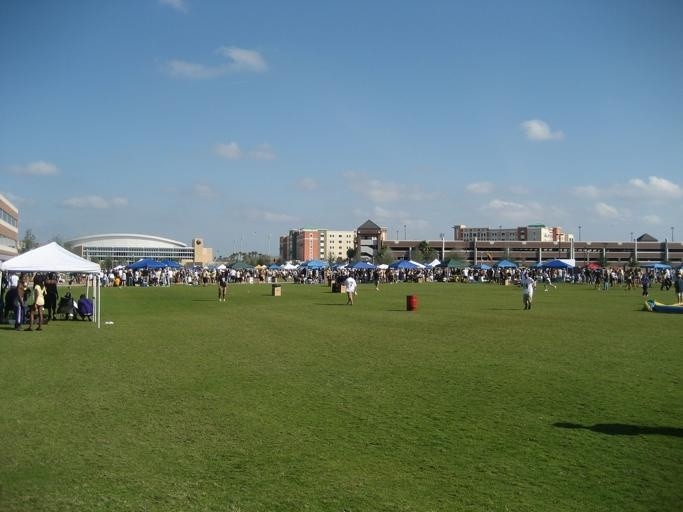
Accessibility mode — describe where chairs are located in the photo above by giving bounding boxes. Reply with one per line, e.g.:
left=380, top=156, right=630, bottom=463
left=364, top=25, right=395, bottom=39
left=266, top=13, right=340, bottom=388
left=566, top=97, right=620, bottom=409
left=58, top=296, right=75, bottom=320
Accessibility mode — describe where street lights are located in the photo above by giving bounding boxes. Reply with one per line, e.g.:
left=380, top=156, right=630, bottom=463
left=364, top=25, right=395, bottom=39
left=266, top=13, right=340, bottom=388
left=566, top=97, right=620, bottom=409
left=556, top=233, right=564, bottom=259
left=579, top=225, right=582, bottom=241
left=630, top=232, right=634, bottom=241
left=404, top=224, right=407, bottom=240
left=670, top=227, right=674, bottom=242
left=396, top=230, right=399, bottom=239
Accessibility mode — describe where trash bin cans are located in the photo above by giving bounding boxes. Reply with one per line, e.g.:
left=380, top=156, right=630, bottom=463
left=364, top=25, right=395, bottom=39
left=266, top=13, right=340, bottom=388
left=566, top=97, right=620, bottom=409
left=272, top=284, right=281, bottom=296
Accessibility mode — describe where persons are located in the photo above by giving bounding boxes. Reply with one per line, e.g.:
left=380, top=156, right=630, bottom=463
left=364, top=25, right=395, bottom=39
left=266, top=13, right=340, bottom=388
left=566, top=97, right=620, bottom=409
left=527, top=266, right=682, bottom=305
left=216, top=271, right=227, bottom=302
left=521, top=273, right=534, bottom=310
left=345, top=273, right=356, bottom=306
left=1, top=272, right=95, bottom=330
left=289, top=267, right=527, bottom=291
left=97, top=266, right=285, bottom=288
